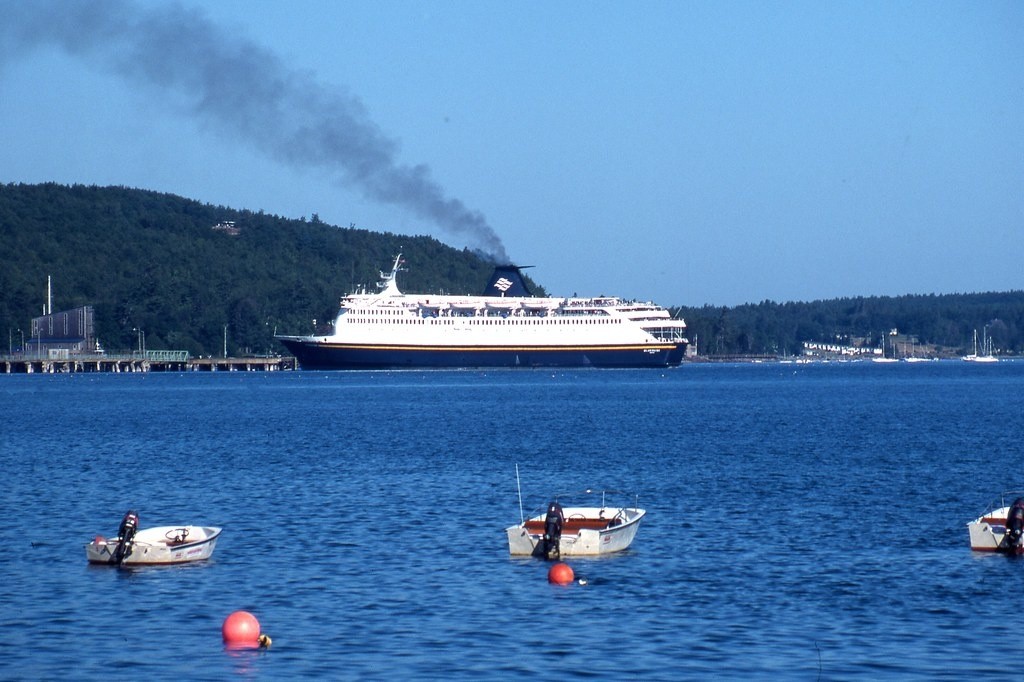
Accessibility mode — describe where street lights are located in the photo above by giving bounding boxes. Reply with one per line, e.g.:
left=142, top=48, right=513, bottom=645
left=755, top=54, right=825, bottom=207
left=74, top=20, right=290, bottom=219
left=133, top=328, right=145, bottom=358
left=18, top=329, right=24, bottom=357
left=35, top=327, right=43, bottom=359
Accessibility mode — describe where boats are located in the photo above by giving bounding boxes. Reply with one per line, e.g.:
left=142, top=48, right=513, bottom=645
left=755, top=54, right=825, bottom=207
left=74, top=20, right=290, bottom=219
left=505, top=487, right=647, bottom=558
left=966, top=486, right=1024, bottom=555
left=272, top=250, right=689, bottom=369
left=84, top=507, right=223, bottom=569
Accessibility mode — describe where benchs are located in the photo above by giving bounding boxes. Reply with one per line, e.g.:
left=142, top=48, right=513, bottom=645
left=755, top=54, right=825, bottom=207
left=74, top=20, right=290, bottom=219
left=980, top=518, right=1006, bottom=525
left=524, top=518, right=622, bottom=534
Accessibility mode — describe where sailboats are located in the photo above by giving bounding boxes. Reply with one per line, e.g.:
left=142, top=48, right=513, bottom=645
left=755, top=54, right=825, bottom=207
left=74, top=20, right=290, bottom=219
left=872, top=330, right=939, bottom=362
left=962, top=327, right=1000, bottom=362
left=752, top=347, right=863, bottom=363
